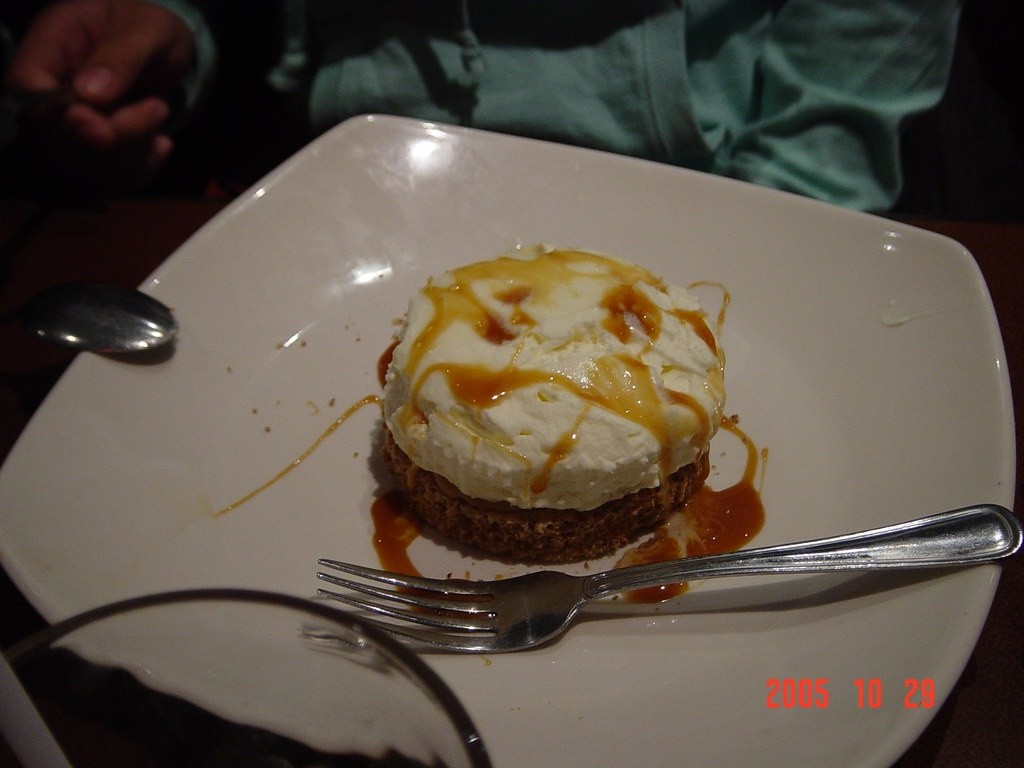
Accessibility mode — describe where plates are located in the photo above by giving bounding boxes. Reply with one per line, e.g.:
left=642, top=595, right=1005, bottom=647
left=0, top=113, right=1014, bottom=766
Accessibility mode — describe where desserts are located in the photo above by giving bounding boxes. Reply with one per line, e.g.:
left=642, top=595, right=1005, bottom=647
left=382, top=245, right=725, bottom=567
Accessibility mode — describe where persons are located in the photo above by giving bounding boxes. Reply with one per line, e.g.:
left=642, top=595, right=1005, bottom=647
left=0, top=0, right=1001, bottom=216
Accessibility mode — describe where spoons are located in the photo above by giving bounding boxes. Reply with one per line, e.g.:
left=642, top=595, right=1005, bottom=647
left=1, top=266, right=179, bottom=353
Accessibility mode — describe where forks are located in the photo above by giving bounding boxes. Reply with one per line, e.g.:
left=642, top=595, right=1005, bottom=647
left=316, top=503, right=1023, bottom=659
